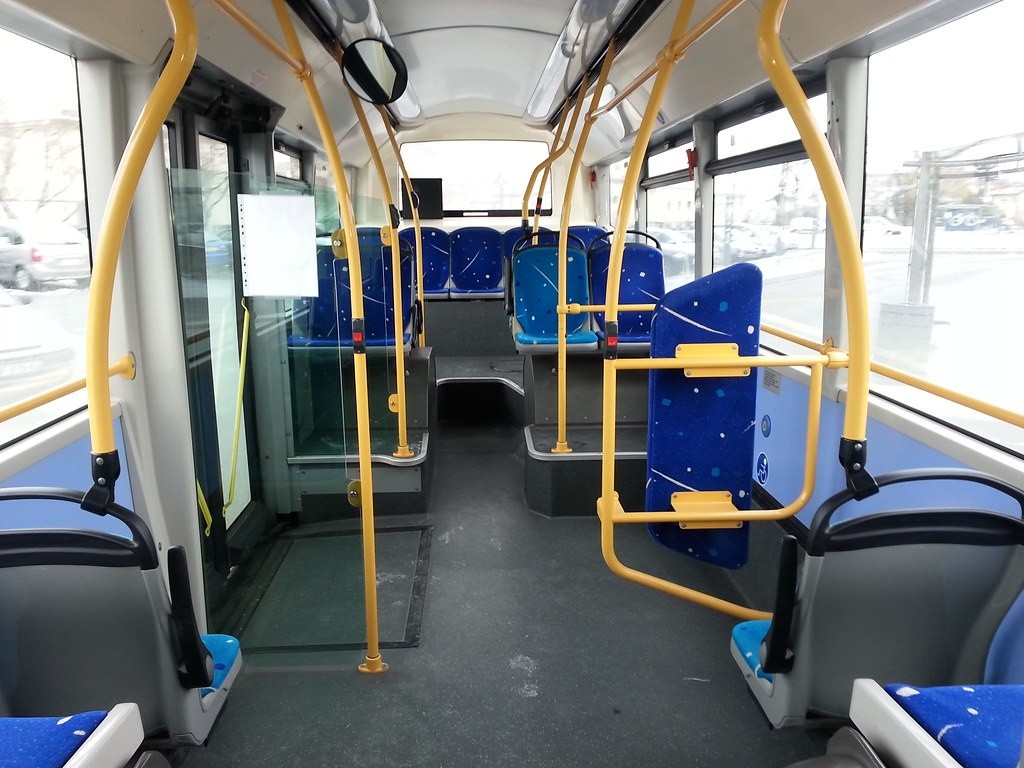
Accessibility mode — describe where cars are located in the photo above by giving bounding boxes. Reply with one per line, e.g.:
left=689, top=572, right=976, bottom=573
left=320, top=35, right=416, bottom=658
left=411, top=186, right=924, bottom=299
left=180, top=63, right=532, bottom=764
left=790, top=217, right=825, bottom=233
left=863, top=216, right=901, bottom=236
left=626, top=212, right=781, bottom=277
left=180, top=228, right=265, bottom=280
left=0, top=218, right=91, bottom=292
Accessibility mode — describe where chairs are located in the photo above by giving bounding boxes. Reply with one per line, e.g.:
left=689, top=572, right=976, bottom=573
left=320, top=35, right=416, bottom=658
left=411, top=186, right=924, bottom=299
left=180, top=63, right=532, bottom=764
left=731, top=467, right=1024, bottom=730
left=790, top=679, right=1024, bottom=768
left=588, top=230, right=665, bottom=352
left=357, top=226, right=610, bottom=299
left=288, top=233, right=416, bottom=358
left=0, top=703, right=172, bottom=768
left=512, top=231, right=599, bottom=352
left=0, top=486, right=241, bottom=750
